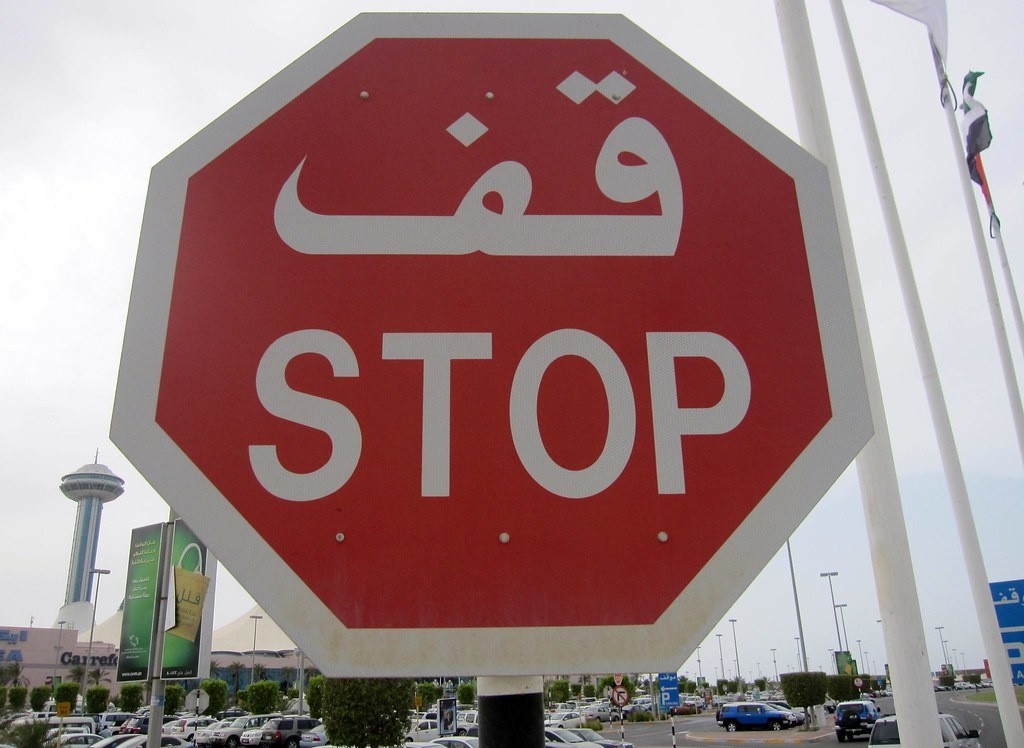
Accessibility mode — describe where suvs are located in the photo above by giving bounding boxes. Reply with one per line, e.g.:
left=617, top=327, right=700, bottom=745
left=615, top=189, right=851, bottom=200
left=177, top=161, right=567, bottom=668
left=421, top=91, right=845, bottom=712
left=834, top=698, right=881, bottom=742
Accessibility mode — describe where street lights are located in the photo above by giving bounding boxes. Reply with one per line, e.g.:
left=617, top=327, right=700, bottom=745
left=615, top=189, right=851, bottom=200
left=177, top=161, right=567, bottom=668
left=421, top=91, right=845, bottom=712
left=716, top=632, right=727, bottom=680
left=794, top=634, right=803, bottom=675
left=729, top=617, right=744, bottom=680
left=756, top=663, right=764, bottom=679
left=768, top=647, right=779, bottom=684
left=695, top=647, right=703, bottom=687
left=934, top=625, right=967, bottom=675
left=819, top=568, right=877, bottom=681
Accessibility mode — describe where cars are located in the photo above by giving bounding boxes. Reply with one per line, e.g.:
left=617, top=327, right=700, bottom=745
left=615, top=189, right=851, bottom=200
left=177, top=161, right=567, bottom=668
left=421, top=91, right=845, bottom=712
left=868, top=711, right=980, bottom=748
left=7, top=680, right=810, bottom=748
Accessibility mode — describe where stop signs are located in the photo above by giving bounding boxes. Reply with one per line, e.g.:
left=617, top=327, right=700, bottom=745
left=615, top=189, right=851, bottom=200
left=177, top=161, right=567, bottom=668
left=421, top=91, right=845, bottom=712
left=110, top=7, right=873, bottom=688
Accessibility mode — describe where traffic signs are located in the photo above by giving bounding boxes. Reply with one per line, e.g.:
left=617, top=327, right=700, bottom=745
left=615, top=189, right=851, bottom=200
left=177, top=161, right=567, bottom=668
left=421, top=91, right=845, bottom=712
left=658, top=675, right=680, bottom=706
